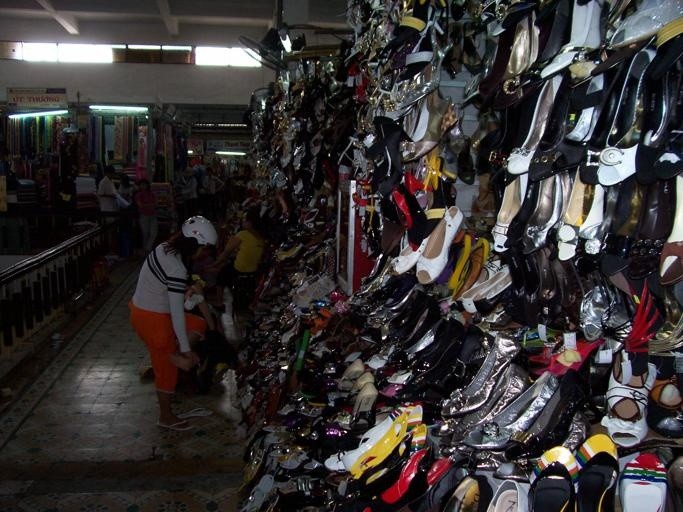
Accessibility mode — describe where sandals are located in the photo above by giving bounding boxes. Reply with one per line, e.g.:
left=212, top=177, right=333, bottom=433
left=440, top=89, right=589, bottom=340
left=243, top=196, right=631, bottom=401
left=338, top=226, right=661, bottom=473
left=415, top=206, right=463, bottom=284
left=448, top=103, right=464, bottom=154
left=387, top=236, right=429, bottom=275
left=461, top=22, right=481, bottom=75
left=457, top=135, right=475, bottom=185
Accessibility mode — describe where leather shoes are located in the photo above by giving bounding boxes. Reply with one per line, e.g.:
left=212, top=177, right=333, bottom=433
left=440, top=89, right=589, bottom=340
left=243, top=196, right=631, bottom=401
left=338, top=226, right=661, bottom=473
left=462, top=371, right=559, bottom=449
left=430, top=398, right=586, bottom=481
left=440, top=333, right=521, bottom=415
left=461, top=264, right=512, bottom=313
left=459, top=362, right=529, bottom=430
left=456, top=260, right=500, bottom=310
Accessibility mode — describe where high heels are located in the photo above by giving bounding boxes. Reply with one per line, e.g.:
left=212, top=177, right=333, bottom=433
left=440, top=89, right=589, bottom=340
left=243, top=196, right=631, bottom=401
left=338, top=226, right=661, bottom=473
left=355, top=71, right=413, bottom=134
left=414, top=454, right=477, bottom=512
left=464, top=74, right=481, bottom=102
left=423, top=155, right=457, bottom=236
left=527, top=79, right=574, bottom=181
left=526, top=445, right=580, bottom=511
left=485, top=479, right=528, bottom=512
left=448, top=235, right=489, bottom=301
left=619, top=452, right=667, bottom=511
left=606, top=354, right=657, bottom=422
left=647, top=276, right=682, bottom=380
left=349, top=404, right=422, bottom=475
left=540, top=0, right=604, bottom=80
left=573, top=435, right=620, bottom=511
left=579, top=284, right=610, bottom=342
left=596, top=50, right=656, bottom=187
left=504, top=177, right=540, bottom=248
left=491, top=173, right=528, bottom=252
left=527, top=1, right=575, bottom=82
left=449, top=0, right=469, bottom=20
left=600, top=273, right=633, bottom=354
left=580, top=348, right=615, bottom=424
left=504, top=368, right=601, bottom=460
left=374, top=317, right=483, bottom=399
left=599, top=175, right=646, bottom=276
left=647, top=367, right=682, bottom=437
left=522, top=174, right=562, bottom=254
left=649, top=374, right=681, bottom=409
left=396, top=0, right=446, bottom=40
left=356, top=432, right=451, bottom=511
left=348, top=253, right=409, bottom=313
left=635, top=36, right=683, bottom=185
left=621, top=272, right=664, bottom=376
left=477, top=3, right=554, bottom=110
left=599, top=404, right=648, bottom=447
left=507, top=251, right=525, bottom=322
left=353, top=423, right=427, bottom=484
left=578, top=49, right=632, bottom=185
left=366, top=275, right=425, bottom=329
left=337, top=358, right=364, bottom=393
left=574, top=184, right=618, bottom=275
left=349, top=382, right=379, bottom=434
left=519, top=253, right=539, bottom=327
left=467, top=1, right=482, bottom=20
left=324, top=449, right=347, bottom=472
left=398, top=90, right=458, bottom=163
left=337, top=133, right=375, bottom=185
left=392, top=170, right=427, bottom=251
left=367, top=318, right=447, bottom=370
left=345, top=371, right=374, bottom=410
left=341, top=401, right=413, bottom=473
left=545, top=170, right=576, bottom=243
left=506, top=75, right=563, bottom=175
left=475, top=108, right=521, bottom=190
left=557, top=184, right=604, bottom=261
left=551, top=74, right=607, bottom=171
left=343, top=0, right=418, bottom=71
left=534, top=243, right=560, bottom=326
left=405, top=7, right=444, bottom=75
left=374, top=116, right=413, bottom=196
left=401, top=28, right=453, bottom=108
left=627, top=176, right=675, bottom=280
left=659, top=172, right=682, bottom=285
left=552, top=256, right=586, bottom=333
left=360, top=290, right=440, bottom=349
left=436, top=473, right=493, bottom=511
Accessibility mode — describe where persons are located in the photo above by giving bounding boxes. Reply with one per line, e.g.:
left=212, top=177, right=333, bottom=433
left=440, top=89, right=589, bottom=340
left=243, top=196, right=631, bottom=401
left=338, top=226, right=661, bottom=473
left=140, top=137, right=148, bottom=166
left=172, top=165, right=249, bottom=211
left=127, top=214, right=217, bottom=431
left=44, top=157, right=158, bottom=251
left=140, top=269, right=241, bottom=388
left=210, top=213, right=265, bottom=313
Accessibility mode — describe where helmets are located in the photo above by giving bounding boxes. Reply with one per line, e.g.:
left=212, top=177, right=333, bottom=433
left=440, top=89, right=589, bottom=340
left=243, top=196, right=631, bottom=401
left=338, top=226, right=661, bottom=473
left=181, top=215, right=218, bottom=246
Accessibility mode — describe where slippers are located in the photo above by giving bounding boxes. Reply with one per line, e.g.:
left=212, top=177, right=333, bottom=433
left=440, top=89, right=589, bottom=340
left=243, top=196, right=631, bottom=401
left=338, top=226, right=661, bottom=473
left=237, top=449, right=264, bottom=492
left=268, top=444, right=309, bottom=469
left=240, top=474, right=278, bottom=511
left=528, top=338, right=602, bottom=376
left=261, top=423, right=295, bottom=443
left=156, top=419, right=192, bottom=430
left=177, top=407, right=212, bottom=418
left=278, top=384, right=352, bottom=441
